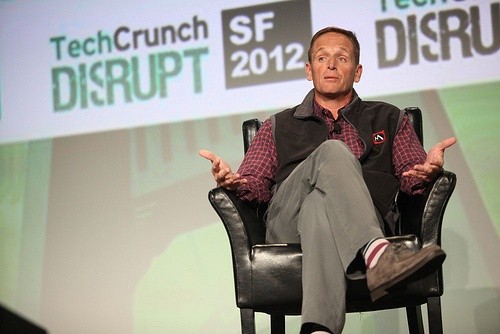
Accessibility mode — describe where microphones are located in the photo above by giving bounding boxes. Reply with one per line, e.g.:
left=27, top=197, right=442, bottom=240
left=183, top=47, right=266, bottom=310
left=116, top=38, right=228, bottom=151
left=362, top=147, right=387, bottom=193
left=334, top=123, right=342, bottom=133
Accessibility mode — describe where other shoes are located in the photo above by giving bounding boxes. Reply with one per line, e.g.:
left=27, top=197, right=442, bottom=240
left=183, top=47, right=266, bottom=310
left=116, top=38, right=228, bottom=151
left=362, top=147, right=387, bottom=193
left=365, top=243, right=446, bottom=304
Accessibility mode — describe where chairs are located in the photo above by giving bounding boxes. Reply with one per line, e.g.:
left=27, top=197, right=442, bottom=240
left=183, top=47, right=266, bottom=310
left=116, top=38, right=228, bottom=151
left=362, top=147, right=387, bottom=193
left=208, top=106, right=459, bottom=334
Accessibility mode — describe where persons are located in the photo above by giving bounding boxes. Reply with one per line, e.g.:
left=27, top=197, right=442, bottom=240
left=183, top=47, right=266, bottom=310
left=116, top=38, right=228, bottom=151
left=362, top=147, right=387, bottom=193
left=198, top=27, right=456, bottom=334
